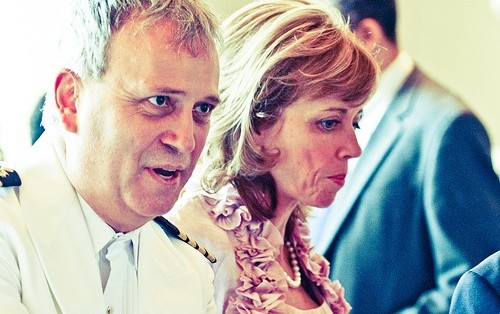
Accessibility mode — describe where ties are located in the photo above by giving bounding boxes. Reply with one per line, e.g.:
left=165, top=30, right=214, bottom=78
left=101, top=239, right=138, bottom=314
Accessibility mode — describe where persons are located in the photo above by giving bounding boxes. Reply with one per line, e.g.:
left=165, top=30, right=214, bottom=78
left=1, top=1, right=222, bottom=314
left=306, top=1, right=499, bottom=314
left=160, top=0, right=381, bottom=314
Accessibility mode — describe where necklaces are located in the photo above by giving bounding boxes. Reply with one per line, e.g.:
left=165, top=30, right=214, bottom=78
left=269, top=233, right=303, bottom=289
